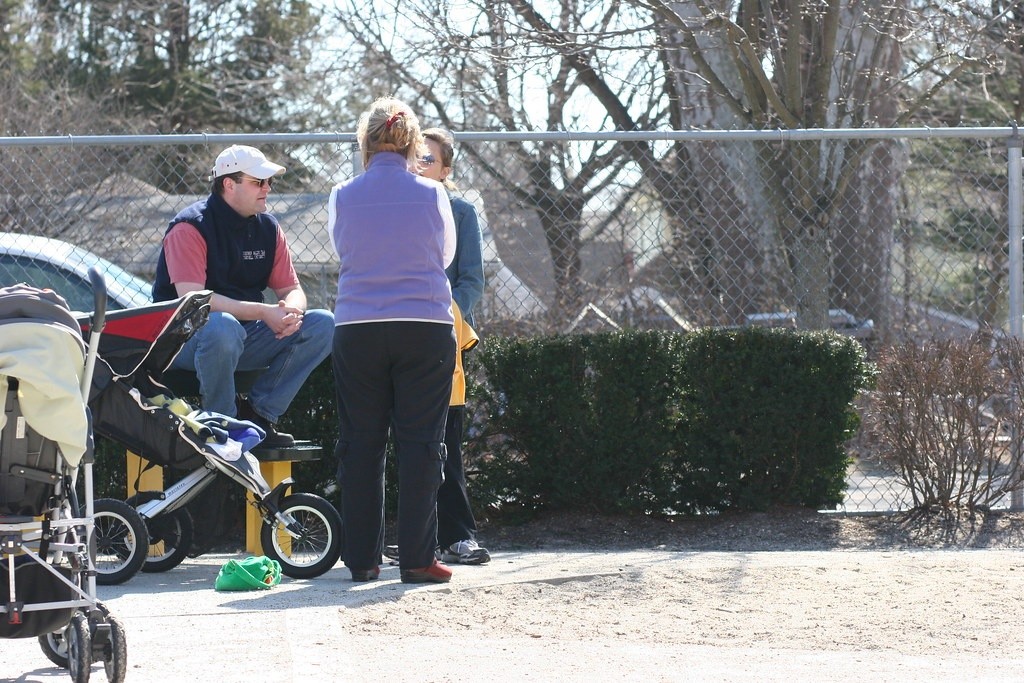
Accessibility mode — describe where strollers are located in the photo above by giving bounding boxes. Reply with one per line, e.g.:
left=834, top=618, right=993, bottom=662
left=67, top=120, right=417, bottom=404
left=0, top=264, right=127, bottom=683
left=69, top=288, right=344, bottom=585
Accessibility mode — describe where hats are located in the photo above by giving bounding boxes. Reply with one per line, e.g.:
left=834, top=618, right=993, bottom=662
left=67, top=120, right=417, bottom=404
left=208, top=144, right=286, bottom=181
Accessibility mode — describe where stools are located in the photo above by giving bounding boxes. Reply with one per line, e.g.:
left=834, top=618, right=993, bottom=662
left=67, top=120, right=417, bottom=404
left=126, top=371, right=323, bottom=559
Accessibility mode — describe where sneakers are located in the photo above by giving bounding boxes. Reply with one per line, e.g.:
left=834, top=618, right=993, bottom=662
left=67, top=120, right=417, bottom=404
left=383, top=545, right=441, bottom=565
left=442, top=540, right=490, bottom=563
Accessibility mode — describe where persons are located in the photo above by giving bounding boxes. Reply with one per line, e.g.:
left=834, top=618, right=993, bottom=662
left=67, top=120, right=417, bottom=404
left=383, top=125, right=492, bottom=566
left=148, top=143, right=336, bottom=446
left=326, top=95, right=459, bottom=584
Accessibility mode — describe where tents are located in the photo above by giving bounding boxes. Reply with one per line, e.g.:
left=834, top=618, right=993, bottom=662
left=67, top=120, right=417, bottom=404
left=0, top=170, right=551, bottom=327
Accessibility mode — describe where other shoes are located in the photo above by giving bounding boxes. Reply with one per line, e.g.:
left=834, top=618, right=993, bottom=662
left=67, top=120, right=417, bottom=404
left=401, top=557, right=451, bottom=583
left=253, top=420, right=294, bottom=448
left=352, top=566, right=380, bottom=582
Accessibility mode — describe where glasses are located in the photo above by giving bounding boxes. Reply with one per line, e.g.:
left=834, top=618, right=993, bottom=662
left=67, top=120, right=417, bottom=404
left=423, top=155, right=441, bottom=165
left=238, top=176, right=273, bottom=187
